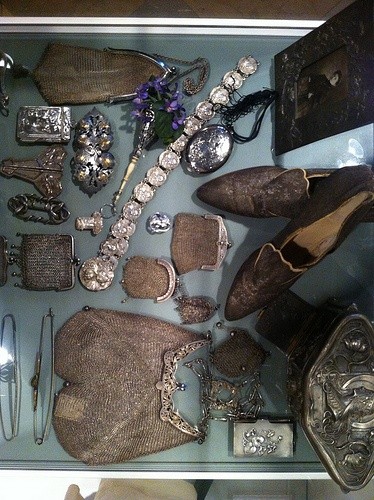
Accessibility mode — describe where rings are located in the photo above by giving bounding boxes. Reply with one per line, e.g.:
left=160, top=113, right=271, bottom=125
left=100, top=202, right=117, bottom=219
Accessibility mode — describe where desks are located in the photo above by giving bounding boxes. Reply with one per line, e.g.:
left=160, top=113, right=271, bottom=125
left=0, top=16, right=374, bottom=500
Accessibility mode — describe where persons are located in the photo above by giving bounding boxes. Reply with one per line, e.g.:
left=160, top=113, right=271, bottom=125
left=297, top=69, right=343, bottom=113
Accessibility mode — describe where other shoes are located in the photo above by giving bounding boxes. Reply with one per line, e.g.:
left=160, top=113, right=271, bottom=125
left=196, top=164, right=374, bottom=321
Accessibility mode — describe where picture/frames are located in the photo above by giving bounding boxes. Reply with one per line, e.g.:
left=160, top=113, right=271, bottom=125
left=275, top=0, right=374, bottom=156
